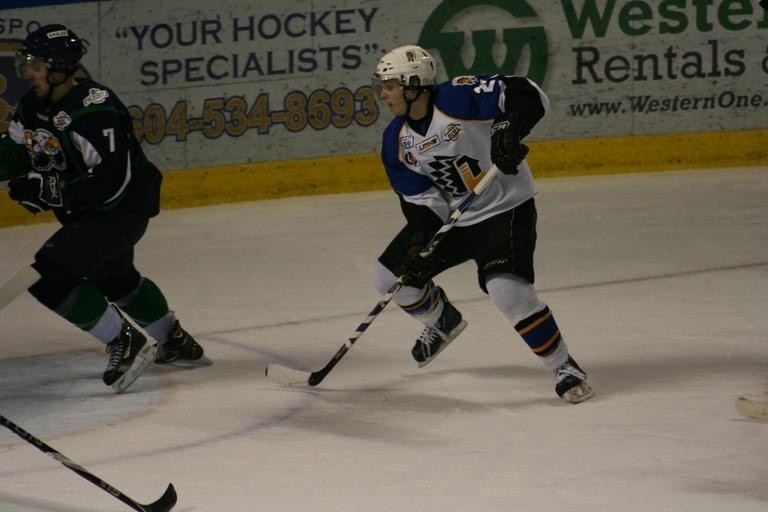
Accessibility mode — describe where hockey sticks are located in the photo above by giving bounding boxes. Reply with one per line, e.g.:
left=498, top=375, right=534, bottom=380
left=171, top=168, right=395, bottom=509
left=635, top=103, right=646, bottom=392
left=265, top=162, right=502, bottom=390
left=0, top=410, right=178, bottom=512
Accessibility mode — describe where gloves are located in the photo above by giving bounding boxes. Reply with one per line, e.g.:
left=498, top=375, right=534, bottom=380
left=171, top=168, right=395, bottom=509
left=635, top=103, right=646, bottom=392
left=489, top=112, right=532, bottom=176
left=397, top=232, right=444, bottom=285
left=7, top=168, right=64, bottom=216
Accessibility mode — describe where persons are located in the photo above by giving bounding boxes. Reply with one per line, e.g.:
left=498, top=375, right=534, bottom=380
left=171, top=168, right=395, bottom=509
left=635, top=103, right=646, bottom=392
left=2, top=24, right=204, bottom=388
left=374, top=45, right=587, bottom=401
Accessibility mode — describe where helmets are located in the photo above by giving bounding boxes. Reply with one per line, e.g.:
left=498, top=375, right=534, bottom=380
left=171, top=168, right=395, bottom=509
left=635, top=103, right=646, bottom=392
left=15, top=22, right=88, bottom=86
left=371, top=45, right=437, bottom=88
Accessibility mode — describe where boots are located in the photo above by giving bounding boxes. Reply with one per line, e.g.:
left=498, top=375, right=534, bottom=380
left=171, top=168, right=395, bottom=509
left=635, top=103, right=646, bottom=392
left=409, top=286, right=462, bottom=362
left=148, top=319, right=204, bottom=364
left=551, top=352, right=587, bottom=396
left=97, top=301, right=147, bottom=386
